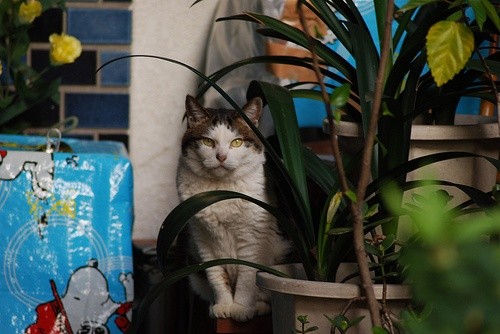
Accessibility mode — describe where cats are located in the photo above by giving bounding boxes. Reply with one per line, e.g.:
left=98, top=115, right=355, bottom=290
left=176, top=94, right=296, bottom=322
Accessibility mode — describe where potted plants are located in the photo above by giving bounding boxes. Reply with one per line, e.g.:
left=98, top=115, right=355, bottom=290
left=95, top=0, right=500, bottom=334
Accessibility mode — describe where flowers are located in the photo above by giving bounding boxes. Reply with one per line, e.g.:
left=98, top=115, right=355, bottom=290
left=0, top=0, right=84, bottom=134
